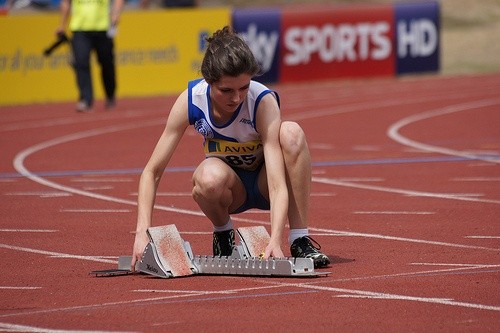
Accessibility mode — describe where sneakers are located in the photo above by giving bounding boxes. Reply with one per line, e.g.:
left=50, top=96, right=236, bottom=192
left=76, top=100, right=90, bottom=111
left=210, top=229, right=235, bottom=258
left=290, top=234, right=330, bottom=268
left=106, top=97, right=116, bottom=109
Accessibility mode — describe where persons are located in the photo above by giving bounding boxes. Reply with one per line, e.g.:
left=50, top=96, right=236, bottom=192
left=57, top=0, right=125, bottom=111
left=130, top=24, right=331, bottom=276
